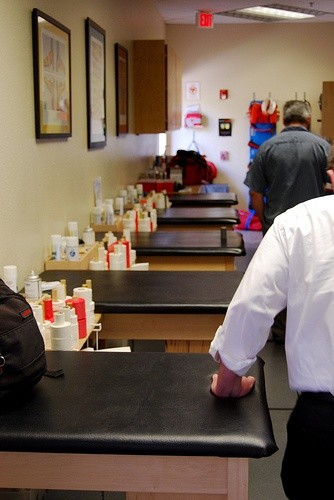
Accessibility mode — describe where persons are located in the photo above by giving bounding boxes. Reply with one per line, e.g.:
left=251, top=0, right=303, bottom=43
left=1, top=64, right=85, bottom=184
left=208, top=142, right=334, bottom=500
left=245, top=99, right=331, bottom=340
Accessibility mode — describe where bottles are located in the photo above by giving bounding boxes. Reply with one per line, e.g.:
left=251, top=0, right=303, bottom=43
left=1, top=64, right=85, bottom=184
left=82, top=225, right=95, bottom=246
left=24, top=270, right=43, bottom=299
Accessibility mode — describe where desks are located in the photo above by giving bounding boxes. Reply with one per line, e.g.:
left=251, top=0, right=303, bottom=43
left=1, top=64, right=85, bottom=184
left=0, top=193, right=280, bottom=500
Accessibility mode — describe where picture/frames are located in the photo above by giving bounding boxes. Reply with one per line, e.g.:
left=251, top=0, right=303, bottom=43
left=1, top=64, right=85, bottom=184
left=114, top=43, right=130, bottom=136
left=31, top=8, right=72, bottom=140
left=85, top=17, right=107, bottom=149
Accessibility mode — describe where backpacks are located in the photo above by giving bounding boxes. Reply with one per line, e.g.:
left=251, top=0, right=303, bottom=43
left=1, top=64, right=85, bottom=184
left=0, top=278, right=66, bottom=395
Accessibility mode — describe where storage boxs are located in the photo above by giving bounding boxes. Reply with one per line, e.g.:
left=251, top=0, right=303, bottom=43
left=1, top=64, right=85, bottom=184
left=140, top=180, right=175, bottom=194
left=93, top=215, right=127, bottom=231
left=46, top=242, right=99, bottom=270
